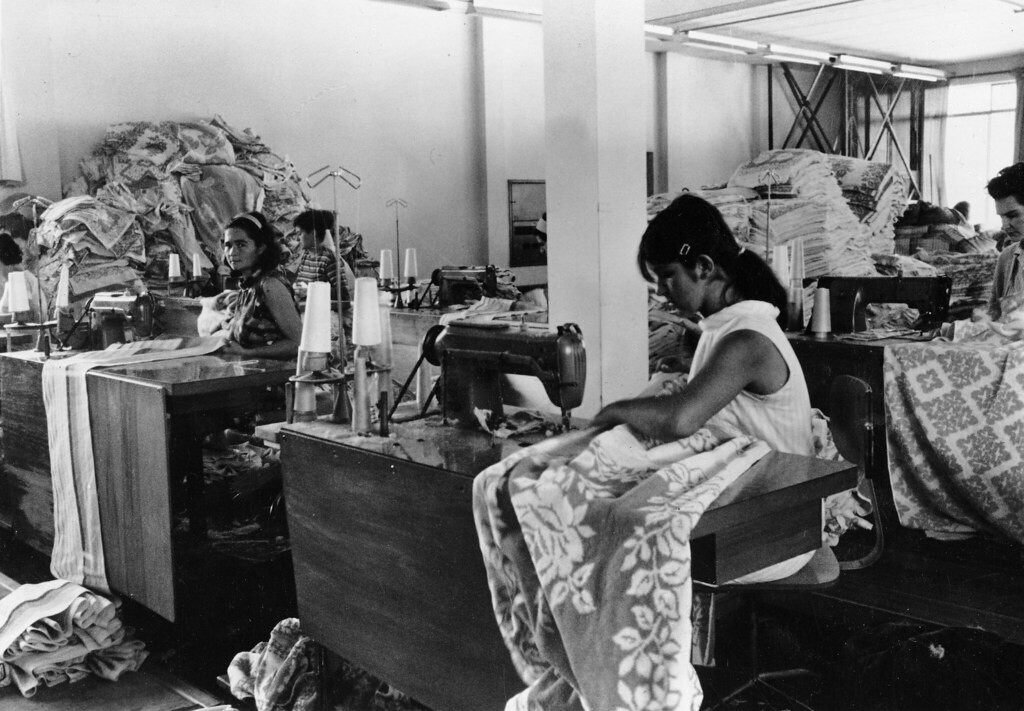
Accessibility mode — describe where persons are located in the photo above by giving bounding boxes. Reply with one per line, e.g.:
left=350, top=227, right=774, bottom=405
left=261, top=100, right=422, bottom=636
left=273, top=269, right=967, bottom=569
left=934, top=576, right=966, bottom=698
left=290, top=209, right=350, bottom=314
left=987, top=162, right=1024, bottom=325
left=218, top=211, right=301, bottom=360
left=588, top=195, right=819, bottom=584
left=0, top=233, right=50, bottom=353
